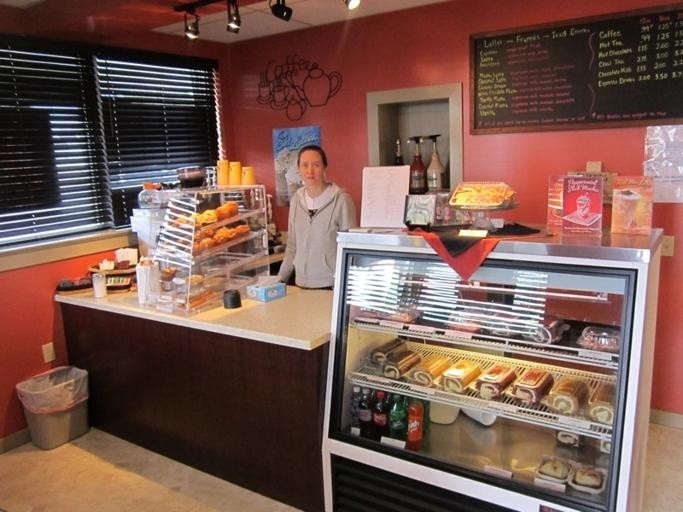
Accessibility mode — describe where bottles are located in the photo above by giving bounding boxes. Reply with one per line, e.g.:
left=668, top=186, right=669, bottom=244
left=350, top=383, right=431, bottom=451
left=134, top=258, right=183, bottom=314
left=395, top=133, right=446, bottom=192
left=138, top=183, right=166, bottom=210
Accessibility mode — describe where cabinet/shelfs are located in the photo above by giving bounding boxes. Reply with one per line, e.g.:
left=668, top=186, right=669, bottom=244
left=314, top=221, right=665, bottom=512
left=140, top=180, right=270, bottom=315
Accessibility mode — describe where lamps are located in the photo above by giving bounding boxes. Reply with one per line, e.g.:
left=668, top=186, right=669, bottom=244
left=171, top=0, right=364, bottom=42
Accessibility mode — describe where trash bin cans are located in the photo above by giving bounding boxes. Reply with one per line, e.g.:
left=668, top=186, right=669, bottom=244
left=17, top=366, right=90, bottom=450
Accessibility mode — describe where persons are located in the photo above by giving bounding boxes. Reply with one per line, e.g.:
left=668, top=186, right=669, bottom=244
left=271, top=145, right=357, bottom=289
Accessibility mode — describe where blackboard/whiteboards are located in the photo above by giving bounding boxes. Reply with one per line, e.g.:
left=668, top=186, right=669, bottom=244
left=470, top=2, right=683, bottom=135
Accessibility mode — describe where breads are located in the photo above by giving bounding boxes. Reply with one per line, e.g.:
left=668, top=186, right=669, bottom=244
left=574, top=467, right=603, bottom=488
left=370, top=339, right=615, bottom=425
left=539, top=458, right=571, bottom=478
left=174, top=202, right=251, bottom=257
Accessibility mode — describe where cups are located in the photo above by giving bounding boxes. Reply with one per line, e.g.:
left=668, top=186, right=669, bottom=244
left=612, top=189, right=641, bottom=232
left=215, top=159, right=257, bottom=186
left=423, top=190, right=488, bottom=226
left=222, top=288, right=242, bottom=308
left=91, top=273, right=108, bottom=297
left=576, top=199, right=589, bottom=221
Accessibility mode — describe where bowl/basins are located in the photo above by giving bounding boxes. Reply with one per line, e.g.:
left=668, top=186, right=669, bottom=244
left=176, top=165, right=207, bottom=188
